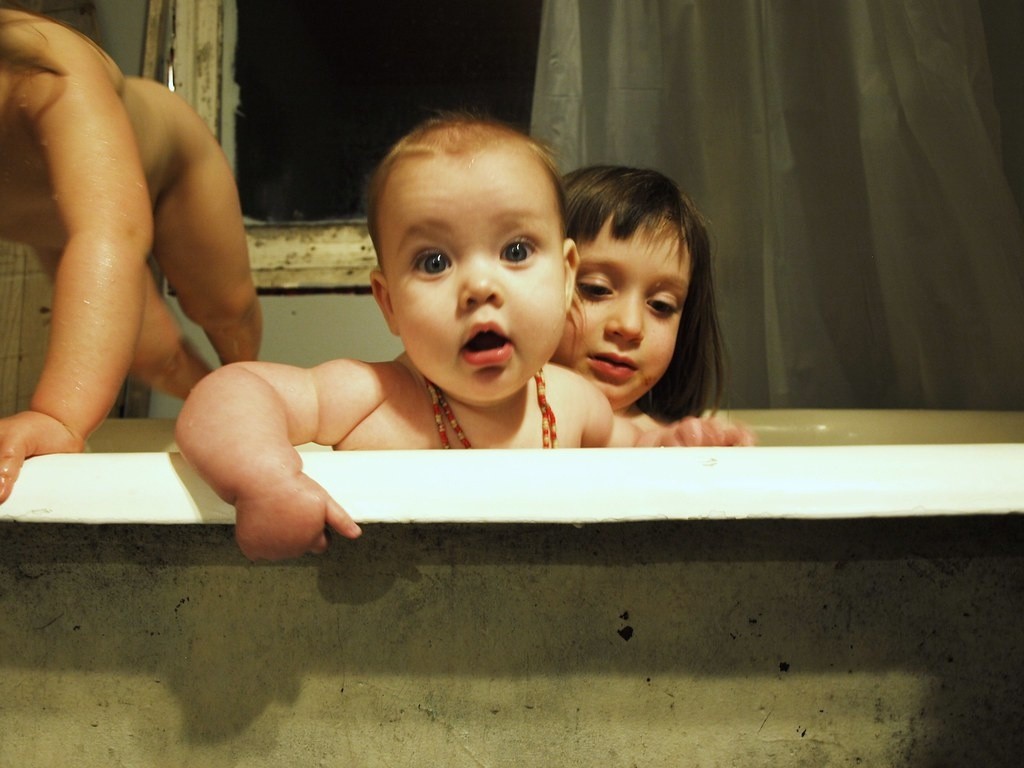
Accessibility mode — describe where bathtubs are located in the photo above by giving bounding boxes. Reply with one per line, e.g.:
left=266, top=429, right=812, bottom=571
left=0, top=442, right=1024, bottom=768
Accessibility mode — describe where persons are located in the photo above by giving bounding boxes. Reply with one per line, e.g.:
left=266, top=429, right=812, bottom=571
left=550, top=165, right=727, bottom=432
left=174, top=119, right=748, bottom=563
left=0, top=7, right=264, bottom=504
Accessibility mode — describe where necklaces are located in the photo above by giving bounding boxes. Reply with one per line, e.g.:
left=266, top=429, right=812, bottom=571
left=424, top=366, right=558, bottom=448
